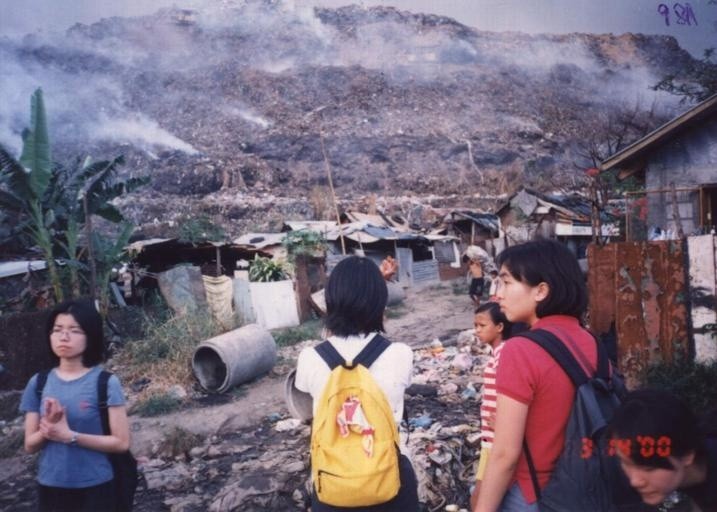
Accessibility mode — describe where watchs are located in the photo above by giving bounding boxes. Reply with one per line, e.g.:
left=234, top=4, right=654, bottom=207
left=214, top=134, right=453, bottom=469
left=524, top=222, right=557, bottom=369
left=71, top=431, right=78, bottom=447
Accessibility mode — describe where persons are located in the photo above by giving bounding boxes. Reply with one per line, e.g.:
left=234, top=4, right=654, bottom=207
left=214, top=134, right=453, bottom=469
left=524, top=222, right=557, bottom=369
left=294, top=256, right=419, bottom=512
left=470, top=303, right=513, bottom=512
left=19, top=301, right=130, bottom=512
left=604, top=390, right=717, bottom=512
left=475, top=241, right=613, bottom=512
left=489, top=270, right=499, bottom=302
left=380, top=253, right=398, bottom=281
left=463, top=255, right=483, bottom=305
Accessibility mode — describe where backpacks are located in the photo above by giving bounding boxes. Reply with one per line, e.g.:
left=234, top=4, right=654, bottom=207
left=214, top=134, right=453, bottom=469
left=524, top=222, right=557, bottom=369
left=36, top=370, right=137, bottom=512
left=512, top=329, right=639, bottom=512
left=311, top=333, right=402, bottom=507
